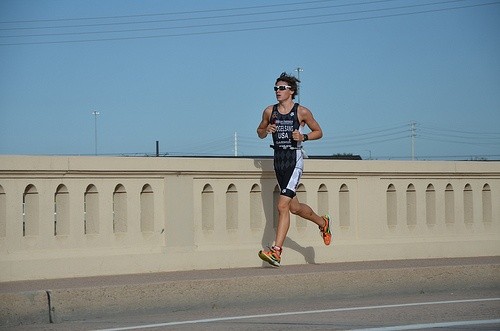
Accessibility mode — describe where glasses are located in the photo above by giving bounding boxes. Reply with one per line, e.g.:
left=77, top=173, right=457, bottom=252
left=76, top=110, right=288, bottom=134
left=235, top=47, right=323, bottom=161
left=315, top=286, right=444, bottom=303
left=274, top=85, right=292, bottom=91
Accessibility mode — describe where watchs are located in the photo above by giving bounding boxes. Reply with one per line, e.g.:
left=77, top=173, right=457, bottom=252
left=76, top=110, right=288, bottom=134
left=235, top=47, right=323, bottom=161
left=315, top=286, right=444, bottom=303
left=302, top=134, right=308, bottom=141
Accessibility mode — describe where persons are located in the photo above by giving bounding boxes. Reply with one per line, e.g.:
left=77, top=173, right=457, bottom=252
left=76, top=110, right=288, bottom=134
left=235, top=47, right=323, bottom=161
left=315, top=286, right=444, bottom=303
left=257, top=71, right=334, bottom=270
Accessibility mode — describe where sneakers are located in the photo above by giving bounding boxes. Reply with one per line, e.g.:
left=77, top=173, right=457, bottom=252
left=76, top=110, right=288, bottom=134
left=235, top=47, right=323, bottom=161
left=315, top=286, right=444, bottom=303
left=319, top=214, right=332, bottom=245
left=258, top=243, right=283, bottom=266
